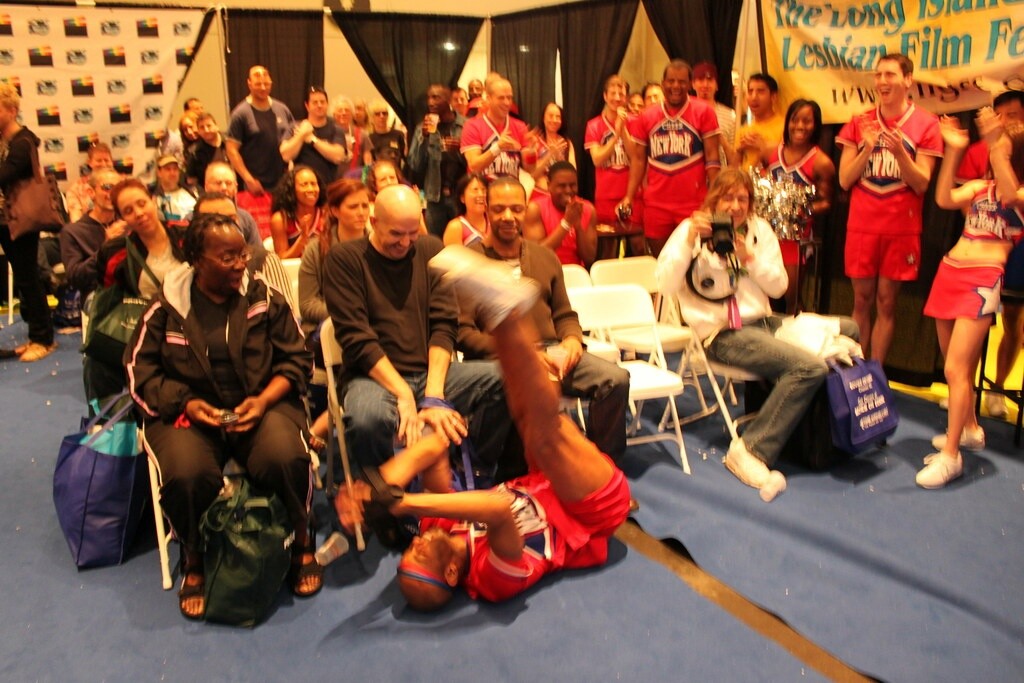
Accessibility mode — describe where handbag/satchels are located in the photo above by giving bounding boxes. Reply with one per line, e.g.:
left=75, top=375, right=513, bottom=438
left=51, top=391, right=141, bottom=570
left=97, top=231, right=161, bottom=345
left=2, top=140, right=65, bottom=241
left=827, top=357, right=901, bottom=447
left=236, top=187, right=274, bottom=240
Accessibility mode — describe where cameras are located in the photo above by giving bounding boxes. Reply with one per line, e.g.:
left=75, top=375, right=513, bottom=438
left=701, top=216, right=735, bottom=256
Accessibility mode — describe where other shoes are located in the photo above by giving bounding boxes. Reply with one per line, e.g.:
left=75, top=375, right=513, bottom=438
left=19, top=342, right=59, bottom=363
left=15, top=337, right=32, bottom=355
left=428, top=245, right=540, bottom=331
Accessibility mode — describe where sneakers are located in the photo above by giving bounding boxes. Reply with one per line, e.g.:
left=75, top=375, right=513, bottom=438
left=915, top=451, right=964, bottom=490
left=725, top=441, right=772, bottom=490
left=932, top=426, right=987, bottom=452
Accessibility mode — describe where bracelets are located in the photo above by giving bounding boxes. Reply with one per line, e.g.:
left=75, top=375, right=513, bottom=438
left=310, top=137, right=317, bottom=146
left=560, top=219, right=570, bottom=231
left=490, top=143, right=501, bottom=157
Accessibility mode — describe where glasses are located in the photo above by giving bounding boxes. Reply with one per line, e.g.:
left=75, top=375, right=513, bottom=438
left=374, top=111, right=386, bottom=117
left=101, top=183, right=111, bottom=191
left=255, top=72, right=269, bottom=78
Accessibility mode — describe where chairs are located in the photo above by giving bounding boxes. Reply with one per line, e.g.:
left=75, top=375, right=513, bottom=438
left=144, top=254, right=765, bottom=591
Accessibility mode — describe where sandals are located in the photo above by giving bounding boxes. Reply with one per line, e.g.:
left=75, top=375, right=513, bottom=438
left=178, top=569, right=206, bottom=620
left=297, top=546, right=326, bottom=597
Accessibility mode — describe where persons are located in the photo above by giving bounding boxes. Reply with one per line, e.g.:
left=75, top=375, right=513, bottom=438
left=56, top=65, right=597, bottom=322
left=587, top=61, right=836, bottom=314
left=915, top=90, right=1024, bottom=489
left=335, top=243, right=630, bottom=607
left=122, top=213, right=323, bottom=617
left=657, top=168, right=829, bottom=488
left=453, top=176, right=630, bottom=463
left=0, top=79, right=59, bottom=361
left=837, top=54, right=943, bottom=365
left=321, top=184, right=510, bottom=492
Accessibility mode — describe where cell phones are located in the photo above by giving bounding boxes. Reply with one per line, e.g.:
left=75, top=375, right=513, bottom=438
left=220, top=412, right=239, bottom=424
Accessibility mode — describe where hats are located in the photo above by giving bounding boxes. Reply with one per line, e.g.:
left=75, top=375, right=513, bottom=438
left=158, top=154, right=178, bottom=167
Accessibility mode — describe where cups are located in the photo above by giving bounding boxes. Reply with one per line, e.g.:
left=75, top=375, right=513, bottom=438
left=315, top=532, right=349, bottom=566
